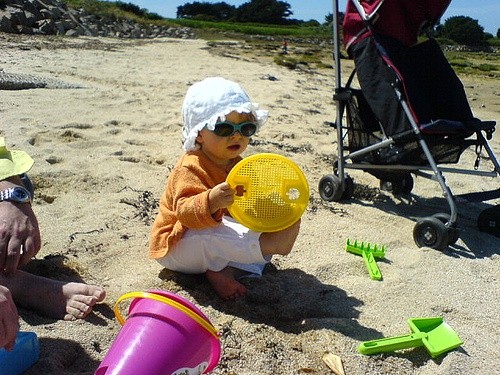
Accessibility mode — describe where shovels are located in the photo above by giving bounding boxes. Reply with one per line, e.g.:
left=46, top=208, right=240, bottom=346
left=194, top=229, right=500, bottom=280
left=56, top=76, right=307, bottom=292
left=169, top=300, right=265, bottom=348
left=359, top=316, right=463, bottom=360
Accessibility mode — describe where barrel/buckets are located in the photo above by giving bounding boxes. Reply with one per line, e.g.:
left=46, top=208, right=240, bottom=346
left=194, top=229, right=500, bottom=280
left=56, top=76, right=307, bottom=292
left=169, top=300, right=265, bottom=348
left=94, top=290, right=221, bottom=375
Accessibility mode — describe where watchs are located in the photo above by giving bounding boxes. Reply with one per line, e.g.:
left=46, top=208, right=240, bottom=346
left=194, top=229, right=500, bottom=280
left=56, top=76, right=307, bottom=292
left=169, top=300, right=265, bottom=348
left=0, top=186, right=33, bottom=208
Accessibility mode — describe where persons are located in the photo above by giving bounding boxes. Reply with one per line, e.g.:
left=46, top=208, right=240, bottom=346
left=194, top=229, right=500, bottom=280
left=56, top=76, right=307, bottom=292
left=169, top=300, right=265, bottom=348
left=149, top=76, right=301, bottom=303
left=0, top=285, right=22, bottom=351
left=0, top=136, right=108, bottom=323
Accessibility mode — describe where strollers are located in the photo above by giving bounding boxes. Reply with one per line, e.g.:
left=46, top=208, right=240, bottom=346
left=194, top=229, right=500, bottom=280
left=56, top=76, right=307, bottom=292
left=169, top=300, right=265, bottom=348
left=318, top=0, right=500, bottom=251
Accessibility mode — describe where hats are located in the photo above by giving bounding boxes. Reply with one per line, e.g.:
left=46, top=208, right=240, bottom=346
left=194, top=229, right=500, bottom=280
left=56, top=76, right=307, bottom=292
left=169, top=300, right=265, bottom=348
left=0, top=137, right=35, bottom=182
left=181, top=76, right=269, bottom=152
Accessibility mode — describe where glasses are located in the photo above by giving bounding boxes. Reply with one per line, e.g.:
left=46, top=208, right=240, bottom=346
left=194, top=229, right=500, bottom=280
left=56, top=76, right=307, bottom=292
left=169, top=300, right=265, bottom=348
left=203, top=121, right=259, bottom=137
